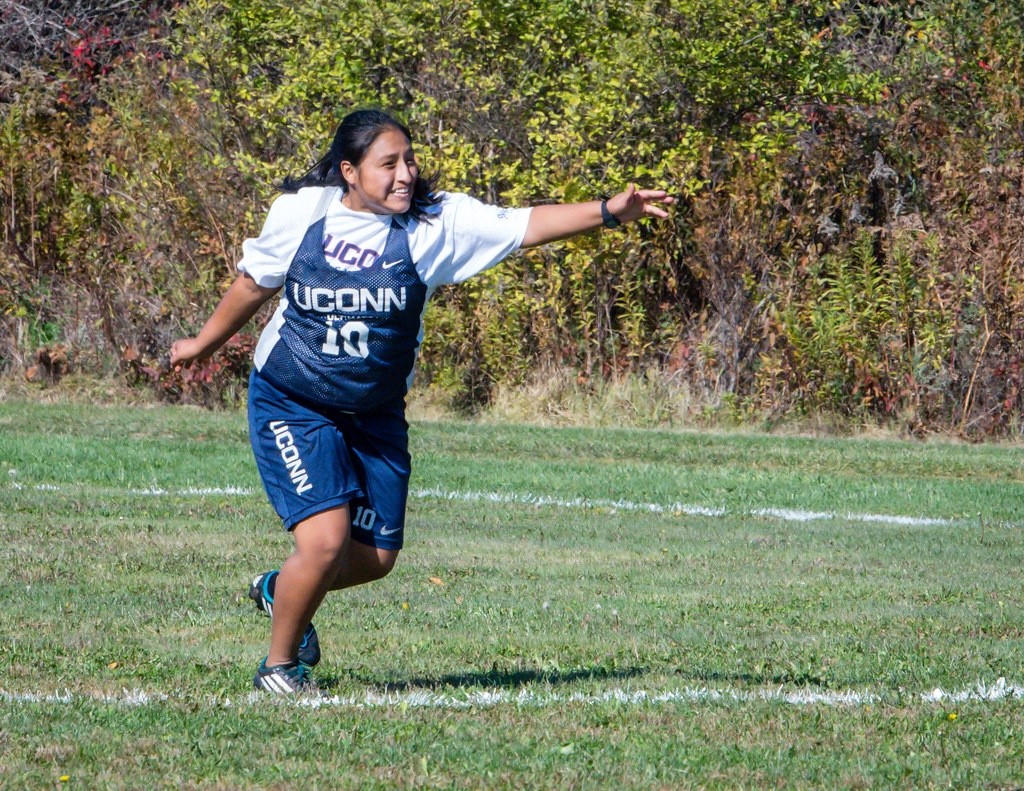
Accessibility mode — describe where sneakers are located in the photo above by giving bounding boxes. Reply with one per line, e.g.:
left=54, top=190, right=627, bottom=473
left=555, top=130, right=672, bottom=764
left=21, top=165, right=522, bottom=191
left=249, top=571, right=321, bottom=667
left=254, top=656, right=326, bottom=700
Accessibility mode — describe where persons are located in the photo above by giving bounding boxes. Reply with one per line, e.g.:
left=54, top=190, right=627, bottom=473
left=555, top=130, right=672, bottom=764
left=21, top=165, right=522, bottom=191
left=170, top=109, right=673, bottom=697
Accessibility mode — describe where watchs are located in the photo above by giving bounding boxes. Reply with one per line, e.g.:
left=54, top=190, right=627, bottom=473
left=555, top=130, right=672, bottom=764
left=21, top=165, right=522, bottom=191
left=600, top=200, right=621, bottom=228
